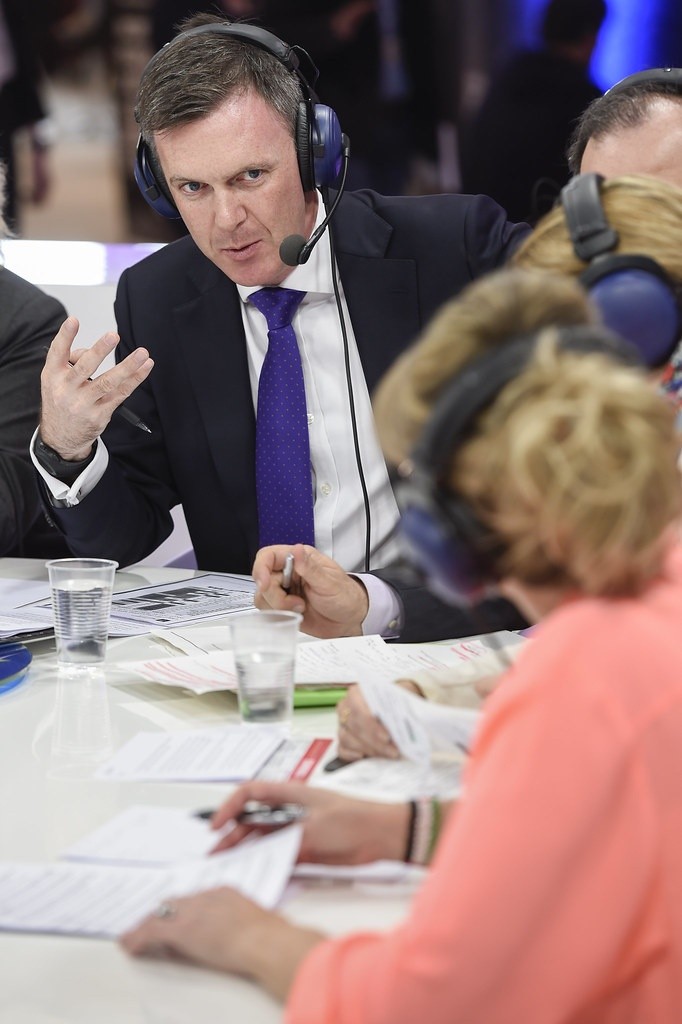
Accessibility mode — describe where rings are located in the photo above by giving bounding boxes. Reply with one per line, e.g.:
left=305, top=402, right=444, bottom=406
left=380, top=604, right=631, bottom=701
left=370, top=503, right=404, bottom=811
left=158, top=904, right=167, bottom=915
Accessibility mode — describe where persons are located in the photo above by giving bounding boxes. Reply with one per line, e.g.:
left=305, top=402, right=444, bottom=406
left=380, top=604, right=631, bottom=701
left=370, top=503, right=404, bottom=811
left=0, top=163, right=70, bottom=558
left=27, top=12, right=532, bottom=579
left=111, top=267, right=682, bottom=1024
left=252, top=1, right=682, bottom=762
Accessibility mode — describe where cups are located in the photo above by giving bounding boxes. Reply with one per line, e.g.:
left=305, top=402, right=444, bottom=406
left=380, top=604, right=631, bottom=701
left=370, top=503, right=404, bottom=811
left=45, top=557, right=118, bottom=666
left=224, top=609, right=303, bottom=726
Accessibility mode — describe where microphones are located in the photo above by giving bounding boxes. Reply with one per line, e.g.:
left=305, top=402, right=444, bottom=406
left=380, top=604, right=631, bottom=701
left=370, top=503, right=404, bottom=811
left=279, top=155, right=348, bottom=266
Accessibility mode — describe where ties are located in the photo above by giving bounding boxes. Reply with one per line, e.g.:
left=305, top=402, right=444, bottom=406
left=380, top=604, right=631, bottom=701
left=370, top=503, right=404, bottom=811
left=248, top=285, right=315, bottom=552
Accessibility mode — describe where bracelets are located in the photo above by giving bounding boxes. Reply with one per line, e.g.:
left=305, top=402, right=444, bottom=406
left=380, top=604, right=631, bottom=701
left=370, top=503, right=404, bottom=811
left=403, top=799, right=440, bottom=866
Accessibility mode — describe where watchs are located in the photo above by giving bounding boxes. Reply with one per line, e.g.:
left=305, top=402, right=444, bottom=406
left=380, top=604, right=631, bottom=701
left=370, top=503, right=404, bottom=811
left=33, top=426, right=98, bottom=481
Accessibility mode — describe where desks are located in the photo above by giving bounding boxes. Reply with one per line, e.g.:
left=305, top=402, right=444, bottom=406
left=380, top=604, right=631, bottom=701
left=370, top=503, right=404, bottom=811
left=0, top=556, right=425, bottom=1024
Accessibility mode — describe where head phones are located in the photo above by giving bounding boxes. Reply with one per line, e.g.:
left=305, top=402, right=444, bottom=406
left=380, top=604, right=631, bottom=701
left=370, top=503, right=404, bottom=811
left=396, top=325, right=637, bottom=609
left=134, top=21, right=351, bottom=221
left=560, top=172, right=681, bottom=369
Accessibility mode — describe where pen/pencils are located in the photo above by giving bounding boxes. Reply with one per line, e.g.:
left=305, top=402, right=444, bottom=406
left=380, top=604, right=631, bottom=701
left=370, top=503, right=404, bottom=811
left=282, top=553, right=294, bottom=592
left=195, top=805, right=302, bottom=823
left=42, top=345, right=152, bottom=433
